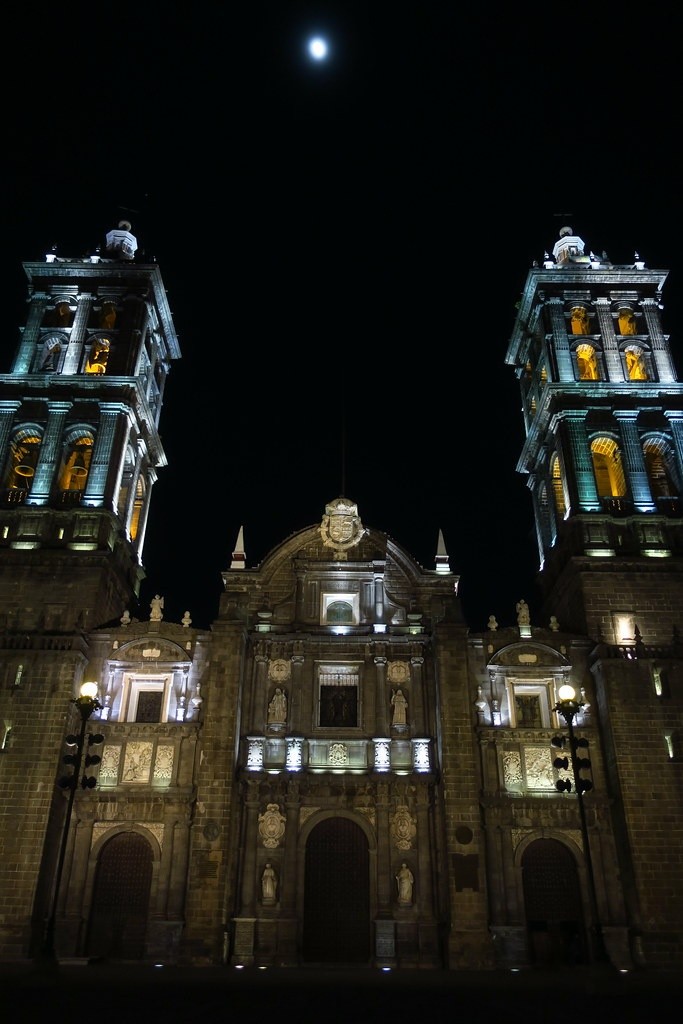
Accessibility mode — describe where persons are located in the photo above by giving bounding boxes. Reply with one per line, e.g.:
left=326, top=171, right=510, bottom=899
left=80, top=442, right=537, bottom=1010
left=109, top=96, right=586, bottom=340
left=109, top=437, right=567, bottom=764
left=272, top=688, right=286, bottom=722
left=391, top=690, right=408, bottom=725
left=261, top=865, right=276, bottom=900
left=517, top=600, right=530, bottom=626
left=150, top=594, right=164, bottom=619
left=395, top=864, right=414, bottom=903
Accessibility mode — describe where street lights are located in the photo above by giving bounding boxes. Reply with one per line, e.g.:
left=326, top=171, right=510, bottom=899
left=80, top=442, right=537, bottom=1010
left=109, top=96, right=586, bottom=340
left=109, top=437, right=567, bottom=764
left=31, top=680, right=104, bottom=965
left=550, top=686, right=607, bottom=966
